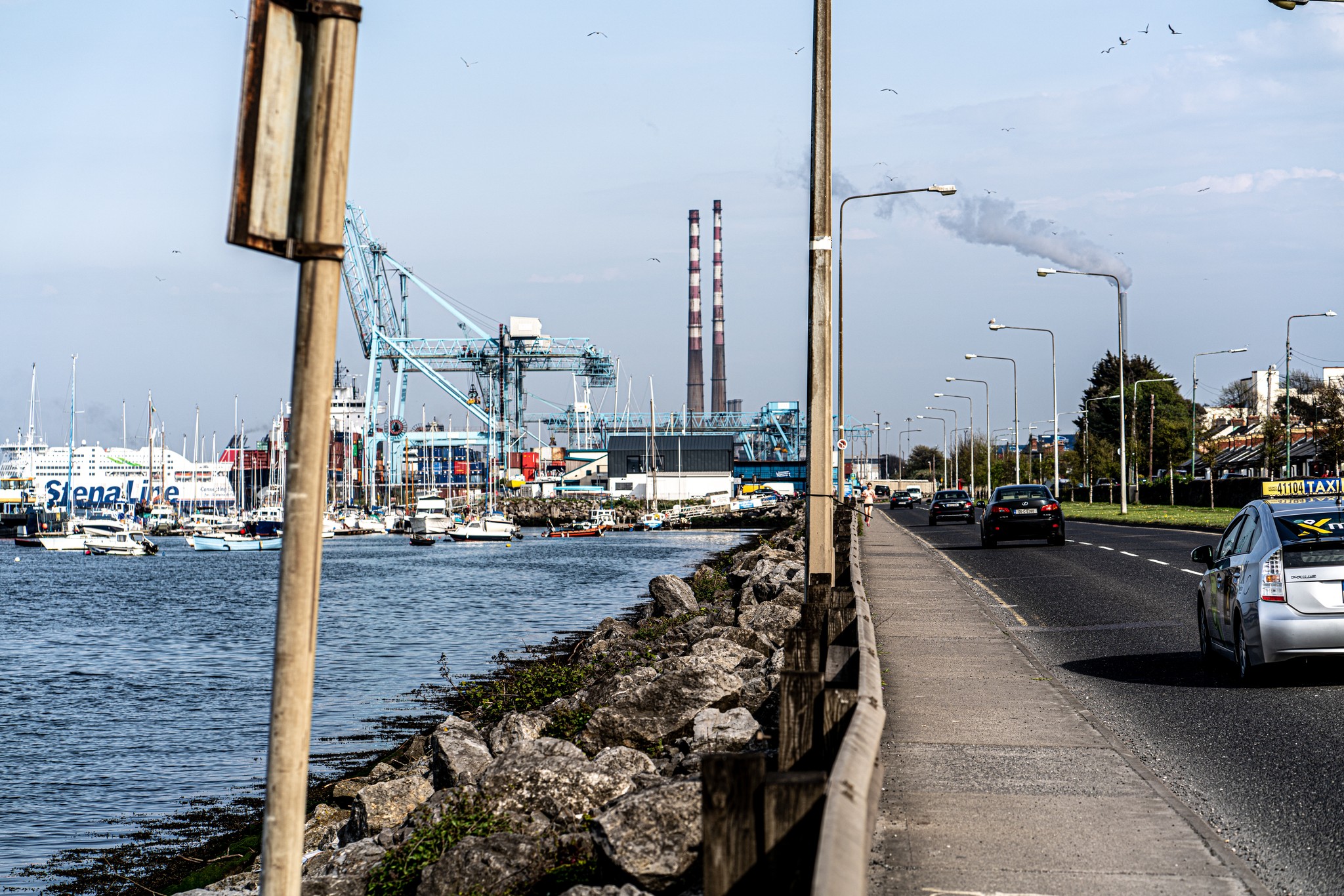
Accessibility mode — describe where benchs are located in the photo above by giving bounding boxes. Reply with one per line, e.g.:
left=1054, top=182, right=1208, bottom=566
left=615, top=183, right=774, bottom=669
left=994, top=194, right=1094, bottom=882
left=1002, top=494, right=1042, bottom=500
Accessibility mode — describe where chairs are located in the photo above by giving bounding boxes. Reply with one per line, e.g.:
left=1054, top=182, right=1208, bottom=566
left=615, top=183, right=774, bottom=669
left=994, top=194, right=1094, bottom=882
left=956, top=493, right=963, bottom=497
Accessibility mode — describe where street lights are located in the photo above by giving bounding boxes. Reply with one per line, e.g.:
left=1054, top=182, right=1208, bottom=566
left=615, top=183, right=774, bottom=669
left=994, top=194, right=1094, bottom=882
left=877, top=411, right=1036, bottom=497
left=1086, top=395, right=1121, bottom=486
left=934, top=393, right=974, bottom=499
left=837, top=184, right=958, bottom=506
left=1028, top=420, right=1055, bottom=484
left=1057, top=410, right=1089, bottom=428
left=1191, top=348, right=1248, bottom=480
left=851, top=422, right=879, bottom=496
left=964, top=353, right=1020, bottom=484
left=899, top=429, right=922, bottom=489
left=1286, top=310, right=1337, bottom=479
left=924, top=407, right=958, bottom=489
left=865, top=427, right=891, bottom=485
left=1037, top=267, right=1126, bottom=514
left=1127, top=377, right=1177, bottom=485
left=989, top=325, right=1059, bottom=499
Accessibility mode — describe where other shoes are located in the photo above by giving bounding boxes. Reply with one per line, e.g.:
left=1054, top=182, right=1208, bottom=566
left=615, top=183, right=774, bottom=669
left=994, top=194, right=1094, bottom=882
left=864, top=517, right=868, bottom=522
left=867, top=523, right=870, bottom=526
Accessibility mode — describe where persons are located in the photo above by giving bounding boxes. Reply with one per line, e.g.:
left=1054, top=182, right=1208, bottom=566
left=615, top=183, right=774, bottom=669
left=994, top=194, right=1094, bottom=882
left=861, top=482, right=877, bottom=525
left=460, top=511, right=465, bottom=528
left=451, top=513, right=455, bottom=524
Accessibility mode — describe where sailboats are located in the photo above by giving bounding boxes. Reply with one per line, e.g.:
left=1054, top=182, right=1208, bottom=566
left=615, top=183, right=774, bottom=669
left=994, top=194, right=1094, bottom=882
left=0, top=354, right=786, bottom=558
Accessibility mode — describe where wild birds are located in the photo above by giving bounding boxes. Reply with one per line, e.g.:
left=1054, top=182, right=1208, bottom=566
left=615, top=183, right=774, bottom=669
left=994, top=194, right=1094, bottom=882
left=1001, top=128, right=1015, bottom=131
left=984, top=189, right=996, bottom=193
left=647, top=258, right=661, bottom=263
left=1203, top=279, right=1207, bottom=280
left=460, top=57, right=478, bottom=67
left=988, top=318, right=996, bottom=324
left=1109, top=234, right=1114, bottom=236
left=1138, top=23, right=1149, bottom=33
left=1198, top=188, right=1210, bottom=192
left=1049, top=221, right=1057, bottom=235
left=1101, top=47, right=1114, bottom=54
left=880, top=89, right=898, bottom=94
left=587, top=32, right=608, bottom=38
left=1119, top=37, right=1131, bottom=45
left=873, top=162, right=897, bottom=181
left=1168, top=24, right=1183, bottom=34
left=155, top=250, right=181, bottom=281
left=230, top=9, right=246, bottom=20
left=1115, top=252, right=1124, bottom=254
left=787, top=47, right=805, bottom=54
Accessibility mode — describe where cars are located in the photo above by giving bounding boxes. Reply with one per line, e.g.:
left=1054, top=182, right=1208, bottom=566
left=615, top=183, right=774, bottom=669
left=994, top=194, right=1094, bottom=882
left=976, top=484, right=1065, bottom=550
left=926, top=489, right=976, bottom=527
left=888, top=491, right=914, bottom=510
left=1045, top=469, right=1322, bottom=480
left=1190, top=477, right=1344, bottom=683
left=853, top=486, right=868, bottom=503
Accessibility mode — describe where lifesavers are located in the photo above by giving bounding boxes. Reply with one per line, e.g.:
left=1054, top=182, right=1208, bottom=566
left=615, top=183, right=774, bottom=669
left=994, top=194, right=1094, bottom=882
left=550, top=492, right=555, bottom=498
left=654, top=514, right=660, bottom=519
left=139, top=520, right=141, bottom=525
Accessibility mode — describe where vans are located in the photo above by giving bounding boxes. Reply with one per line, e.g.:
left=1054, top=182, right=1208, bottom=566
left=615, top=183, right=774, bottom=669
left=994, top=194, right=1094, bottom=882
left=906, top=485, right=923, bottom=505
left=874, top=484, right=892, bottom=497
